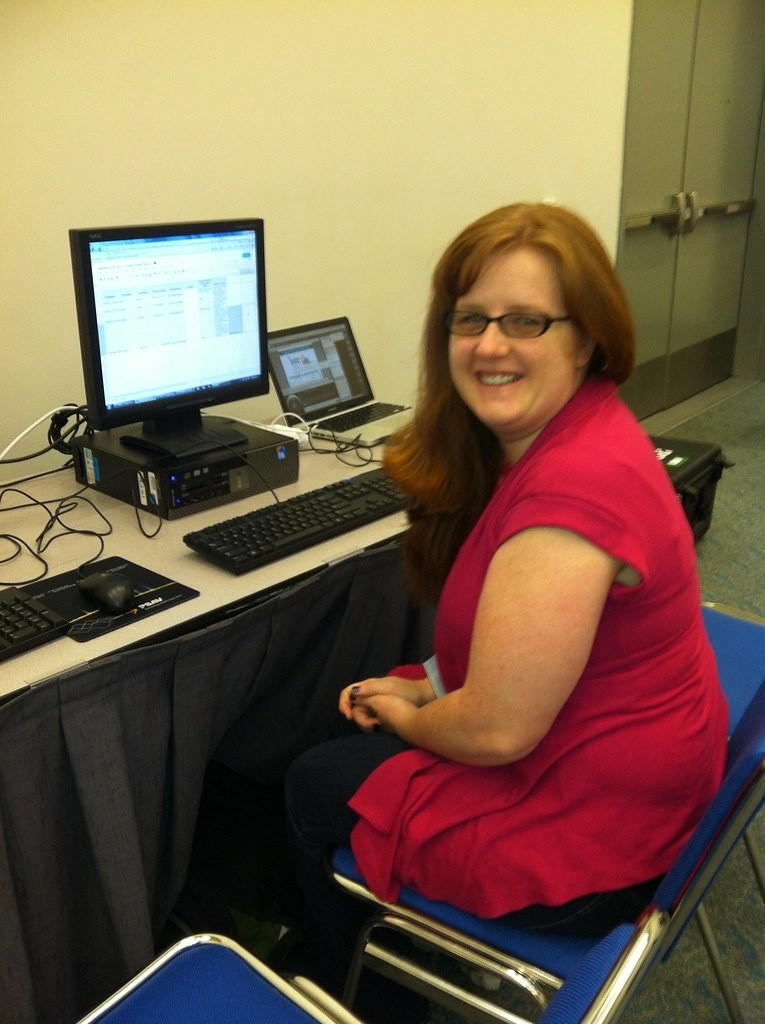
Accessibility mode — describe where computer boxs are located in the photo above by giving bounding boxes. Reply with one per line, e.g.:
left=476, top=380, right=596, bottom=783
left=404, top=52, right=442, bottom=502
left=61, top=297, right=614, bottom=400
left=70, top=414, right=299, bottom=522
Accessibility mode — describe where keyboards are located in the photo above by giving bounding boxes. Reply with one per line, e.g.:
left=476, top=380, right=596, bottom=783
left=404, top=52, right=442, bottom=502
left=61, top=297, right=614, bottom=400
left=0, top=586, right=71, bottom=661
left=182, top=467, right=412, bottom=577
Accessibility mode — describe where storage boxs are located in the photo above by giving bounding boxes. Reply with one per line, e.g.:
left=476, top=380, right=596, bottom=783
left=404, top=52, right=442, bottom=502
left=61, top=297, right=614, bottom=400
left=645, top=433, right=736, bottom=546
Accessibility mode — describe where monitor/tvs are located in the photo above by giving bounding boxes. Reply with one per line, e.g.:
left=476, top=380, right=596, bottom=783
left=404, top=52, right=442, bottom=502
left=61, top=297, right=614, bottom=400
left=69, top=216, right=269, bottom=460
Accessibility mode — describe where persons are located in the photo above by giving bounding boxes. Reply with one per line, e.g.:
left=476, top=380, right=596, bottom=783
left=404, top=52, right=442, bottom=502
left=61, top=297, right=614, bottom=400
left=284, top=203, right=725, bottom=1024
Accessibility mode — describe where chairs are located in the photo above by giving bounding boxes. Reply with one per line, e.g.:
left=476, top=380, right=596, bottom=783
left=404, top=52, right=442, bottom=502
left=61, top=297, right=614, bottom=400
left=73, top=600, right=765, bottom=1024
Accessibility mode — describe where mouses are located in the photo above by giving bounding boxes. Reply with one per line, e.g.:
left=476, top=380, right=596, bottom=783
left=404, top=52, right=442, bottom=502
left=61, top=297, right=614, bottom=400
left=78, top=572, right=134, bottom=616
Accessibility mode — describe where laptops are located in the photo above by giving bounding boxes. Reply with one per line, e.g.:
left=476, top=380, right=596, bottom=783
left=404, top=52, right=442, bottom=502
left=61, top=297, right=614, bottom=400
left=266, top=316, right=417, bottom=447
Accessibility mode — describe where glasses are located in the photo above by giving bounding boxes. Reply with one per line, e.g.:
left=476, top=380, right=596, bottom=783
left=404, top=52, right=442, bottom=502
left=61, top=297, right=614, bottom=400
left=442, top=309, right=573, bottom=338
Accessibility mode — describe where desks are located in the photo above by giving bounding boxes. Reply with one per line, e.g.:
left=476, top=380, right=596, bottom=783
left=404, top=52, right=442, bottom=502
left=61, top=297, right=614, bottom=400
left=0, top=378, right=428, bottom=1024
